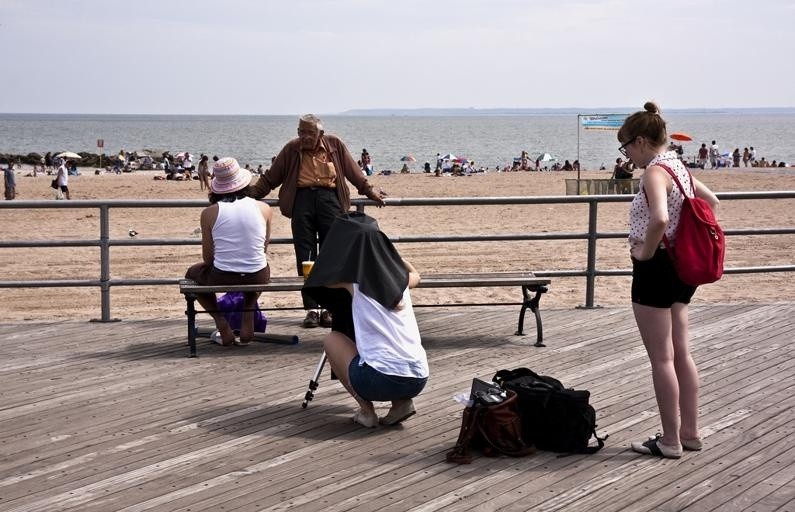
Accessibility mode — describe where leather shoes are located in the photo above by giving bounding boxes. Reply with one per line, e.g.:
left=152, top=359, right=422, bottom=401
left=304, top=311, right=319, bottom=327
left=320, top=310, right=333, bottom=326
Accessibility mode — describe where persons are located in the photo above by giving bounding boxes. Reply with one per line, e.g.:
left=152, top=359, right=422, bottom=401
left=3, top=159, right=15, bottom=200
left=1, top=138, right=276, bottom=180
left=315, top=255, right=431, bottom=430
left=617, top=98, right=720, bottom=458
left=51, top=160, right=74, bottom=200
left=610, top=141, right=785, bottom=178
left=183, top=155, right=271, bottom=347
left=245, top=111, right=389, bottom=331
left=357, top=148, right=581, bottom=179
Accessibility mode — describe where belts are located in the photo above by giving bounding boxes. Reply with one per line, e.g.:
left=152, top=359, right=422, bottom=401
left=297, top=186, right=327, bottom=190
left=213, top=266, right=255, bottom=277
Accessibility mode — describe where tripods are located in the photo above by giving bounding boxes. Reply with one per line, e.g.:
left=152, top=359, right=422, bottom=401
left=300, top=330, right=331, bottom=410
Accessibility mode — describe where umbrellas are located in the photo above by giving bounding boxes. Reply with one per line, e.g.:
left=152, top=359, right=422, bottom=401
left=669, top=133, right=694, bottom=141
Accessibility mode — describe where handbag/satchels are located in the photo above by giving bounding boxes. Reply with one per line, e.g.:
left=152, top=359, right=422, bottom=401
left=492, top=367, right=609, bottom=458
left=51, top=179, right=59, bottom=188
left=447, top=378, right=534, bottom=463
left=217, top=291, right=267, bottom=332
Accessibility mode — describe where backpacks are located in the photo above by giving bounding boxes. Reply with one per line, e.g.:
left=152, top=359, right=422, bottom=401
left=643, top=163, right=725, bottom=286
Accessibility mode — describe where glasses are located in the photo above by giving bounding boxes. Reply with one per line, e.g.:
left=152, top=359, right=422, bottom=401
left=619, top=134, right=644, bottom=155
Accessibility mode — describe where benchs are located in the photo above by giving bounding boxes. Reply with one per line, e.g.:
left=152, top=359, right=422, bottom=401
left=180, top=271, right=551, bottom=358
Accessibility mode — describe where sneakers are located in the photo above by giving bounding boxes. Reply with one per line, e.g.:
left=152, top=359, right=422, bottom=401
left=648, top=435, right=702, bottom=450
left=632, top=433, right=682, bottom=459
left=379, top=398, right=416, bottom=425
left=353, top=408, right=379, bottom=427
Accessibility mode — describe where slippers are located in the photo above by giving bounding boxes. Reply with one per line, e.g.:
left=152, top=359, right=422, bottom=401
left=211, top=330, right=234, bottom=346
left=232, top=329, right=250, bottom=346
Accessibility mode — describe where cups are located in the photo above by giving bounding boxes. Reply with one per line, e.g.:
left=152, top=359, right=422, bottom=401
left=302, top=261, right=314, bottom=281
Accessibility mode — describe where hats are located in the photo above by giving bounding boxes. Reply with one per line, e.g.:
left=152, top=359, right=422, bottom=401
left=210, top=158, right=251, bottom=194
